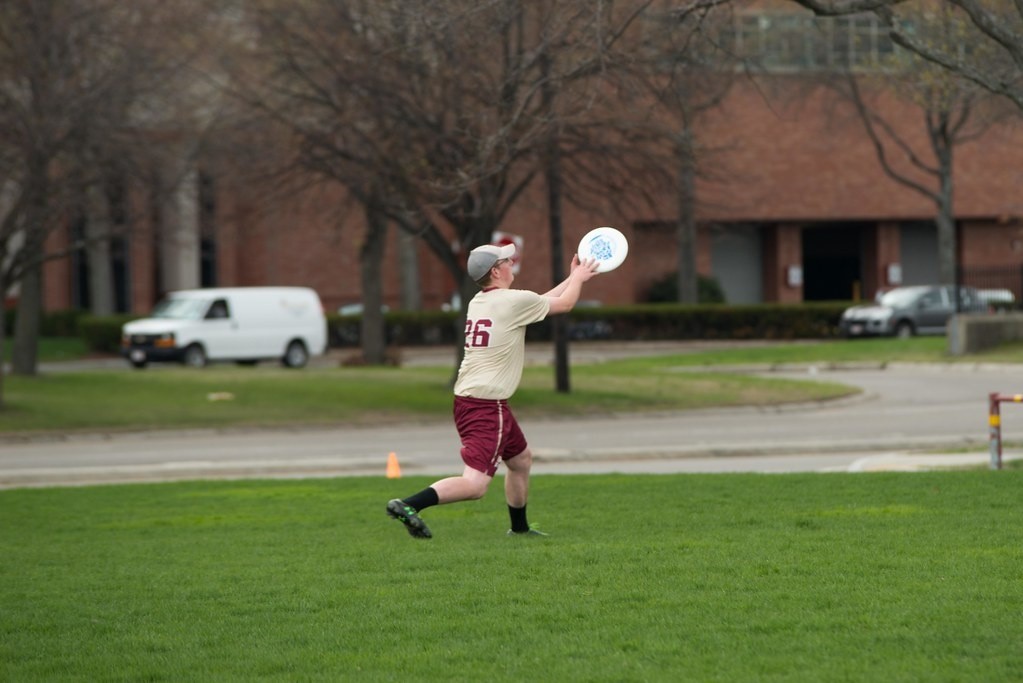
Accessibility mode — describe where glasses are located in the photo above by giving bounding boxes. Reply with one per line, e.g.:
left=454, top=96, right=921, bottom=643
left=488, top=258, right=509, bottom=275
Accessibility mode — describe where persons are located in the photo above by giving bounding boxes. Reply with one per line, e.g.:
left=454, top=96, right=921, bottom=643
left=386, top=244, right=601, bottom=540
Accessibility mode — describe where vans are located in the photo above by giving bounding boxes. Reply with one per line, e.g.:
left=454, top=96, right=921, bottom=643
left=121, top=286, right=329, bottom=370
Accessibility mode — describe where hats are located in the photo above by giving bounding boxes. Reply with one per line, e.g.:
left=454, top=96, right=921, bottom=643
left=467, top=243, right=515, bottom=281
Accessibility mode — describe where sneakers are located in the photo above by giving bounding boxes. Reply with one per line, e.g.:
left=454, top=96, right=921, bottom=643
left=507, top=528, right=549, bottom=536
left=386, top=499, right=432, bottom=538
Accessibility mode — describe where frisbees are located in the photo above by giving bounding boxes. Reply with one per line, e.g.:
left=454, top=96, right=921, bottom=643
left=577, top=226, right=629, bottom=275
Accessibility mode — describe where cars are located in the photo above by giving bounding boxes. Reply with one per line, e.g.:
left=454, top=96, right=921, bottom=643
left=839, top=284, right=984, bottom=338
